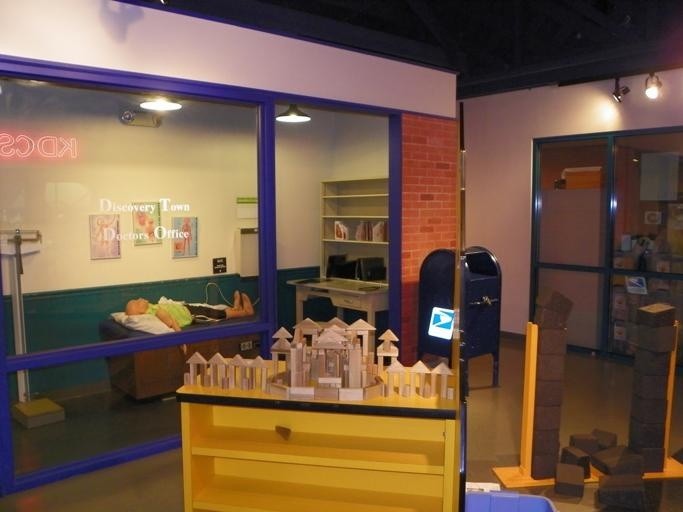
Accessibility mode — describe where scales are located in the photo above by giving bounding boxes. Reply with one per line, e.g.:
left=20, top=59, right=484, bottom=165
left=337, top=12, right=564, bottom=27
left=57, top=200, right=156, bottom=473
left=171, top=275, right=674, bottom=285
left=0, top=229, right=65, bottom=429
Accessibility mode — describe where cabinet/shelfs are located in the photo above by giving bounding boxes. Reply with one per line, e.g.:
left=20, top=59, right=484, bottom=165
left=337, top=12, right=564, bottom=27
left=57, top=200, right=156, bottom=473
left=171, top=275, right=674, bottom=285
left=168, top=352, right=466, bottom=511
left=319, top=175, right=391, bottom=286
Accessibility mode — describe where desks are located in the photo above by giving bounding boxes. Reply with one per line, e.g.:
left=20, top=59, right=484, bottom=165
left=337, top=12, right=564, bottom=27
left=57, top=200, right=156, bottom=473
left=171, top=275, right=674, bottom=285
left=284, top=277, right=390, bottom=364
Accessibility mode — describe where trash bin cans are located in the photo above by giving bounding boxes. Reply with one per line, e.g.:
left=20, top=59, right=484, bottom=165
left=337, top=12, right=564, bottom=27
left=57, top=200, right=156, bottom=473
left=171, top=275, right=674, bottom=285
left=416, top=246, right=501, bottom=397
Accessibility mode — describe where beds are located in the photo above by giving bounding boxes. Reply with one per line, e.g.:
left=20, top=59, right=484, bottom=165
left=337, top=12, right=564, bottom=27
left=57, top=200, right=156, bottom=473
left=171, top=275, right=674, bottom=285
left=95, top=301, right=267, bottom=401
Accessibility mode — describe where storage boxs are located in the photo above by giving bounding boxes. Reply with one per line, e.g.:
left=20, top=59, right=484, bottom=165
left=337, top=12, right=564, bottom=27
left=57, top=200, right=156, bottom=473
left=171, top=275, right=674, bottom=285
left=462, top=491, right=559, bottom=512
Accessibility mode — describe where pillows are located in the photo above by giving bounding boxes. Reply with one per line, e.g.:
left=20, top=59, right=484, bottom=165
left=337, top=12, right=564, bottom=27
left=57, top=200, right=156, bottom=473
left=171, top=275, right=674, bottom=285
left=107, top=309, right=178, bottom=338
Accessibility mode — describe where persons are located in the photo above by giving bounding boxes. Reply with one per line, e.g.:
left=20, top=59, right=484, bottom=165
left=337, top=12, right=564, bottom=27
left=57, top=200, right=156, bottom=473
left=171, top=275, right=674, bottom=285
left=124, top=287, right=255, bottom=349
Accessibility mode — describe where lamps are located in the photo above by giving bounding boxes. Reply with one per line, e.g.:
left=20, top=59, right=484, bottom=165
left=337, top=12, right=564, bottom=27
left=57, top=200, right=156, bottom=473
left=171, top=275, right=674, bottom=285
left=552, top=61, right=683, bottom=107
left=133, top=93, right=183, bottom=113
left=273, top=104, right=314, bottom=125
